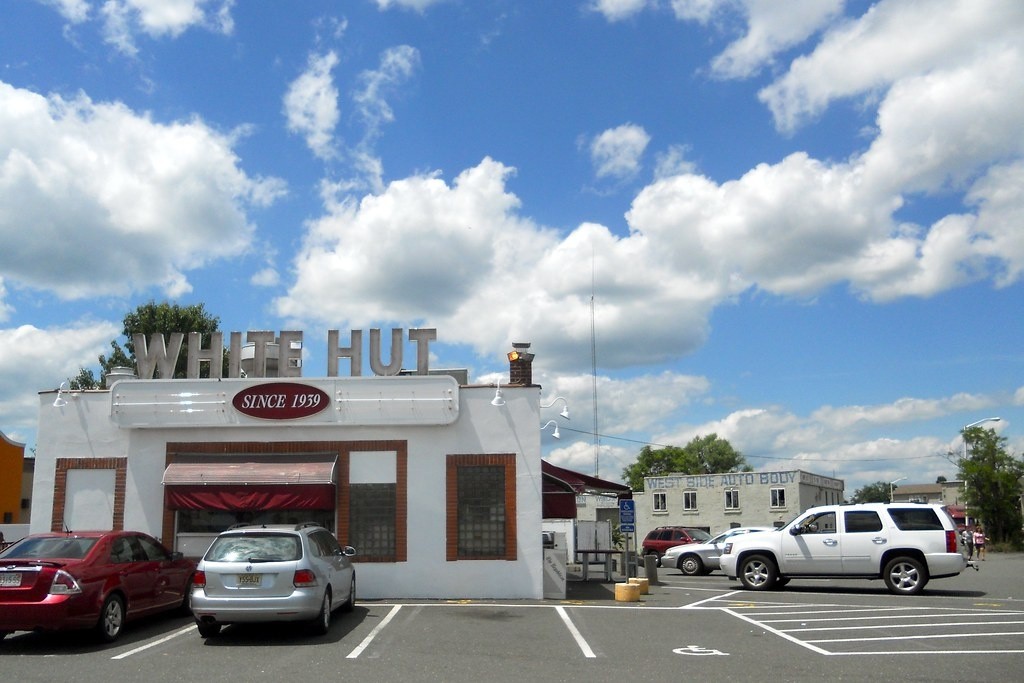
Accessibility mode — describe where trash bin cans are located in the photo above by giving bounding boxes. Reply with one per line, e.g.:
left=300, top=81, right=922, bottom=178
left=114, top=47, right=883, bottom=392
left=642, top=555, right=658, bottom=585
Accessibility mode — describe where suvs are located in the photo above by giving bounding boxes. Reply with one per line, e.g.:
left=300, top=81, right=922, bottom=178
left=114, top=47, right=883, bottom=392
left=718, top=500, right=970, bottom=597
left=641, top=526, right=714, bottom=568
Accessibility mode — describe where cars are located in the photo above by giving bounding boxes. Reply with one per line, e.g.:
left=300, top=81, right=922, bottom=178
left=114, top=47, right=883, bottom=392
left=658, top=526, right=780, bottom=576
left=0, top=528, right=198, bottom=643
left=189, top=521, right=356, bottom=638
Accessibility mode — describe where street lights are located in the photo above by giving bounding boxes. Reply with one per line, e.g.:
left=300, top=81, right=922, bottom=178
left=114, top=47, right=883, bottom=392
left=964, top=416, right=1001, bottom=530
left=890, top=477, right=908, bottom=502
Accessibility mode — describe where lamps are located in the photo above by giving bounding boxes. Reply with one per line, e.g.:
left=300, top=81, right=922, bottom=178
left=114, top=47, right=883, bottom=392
left=541, top=396, right=570, bottom=420
left=490, top=379, right=505, bottom=406
left=53, top=382, right=77, bottom=407
left=540, top=420, right=560, bottom=440
